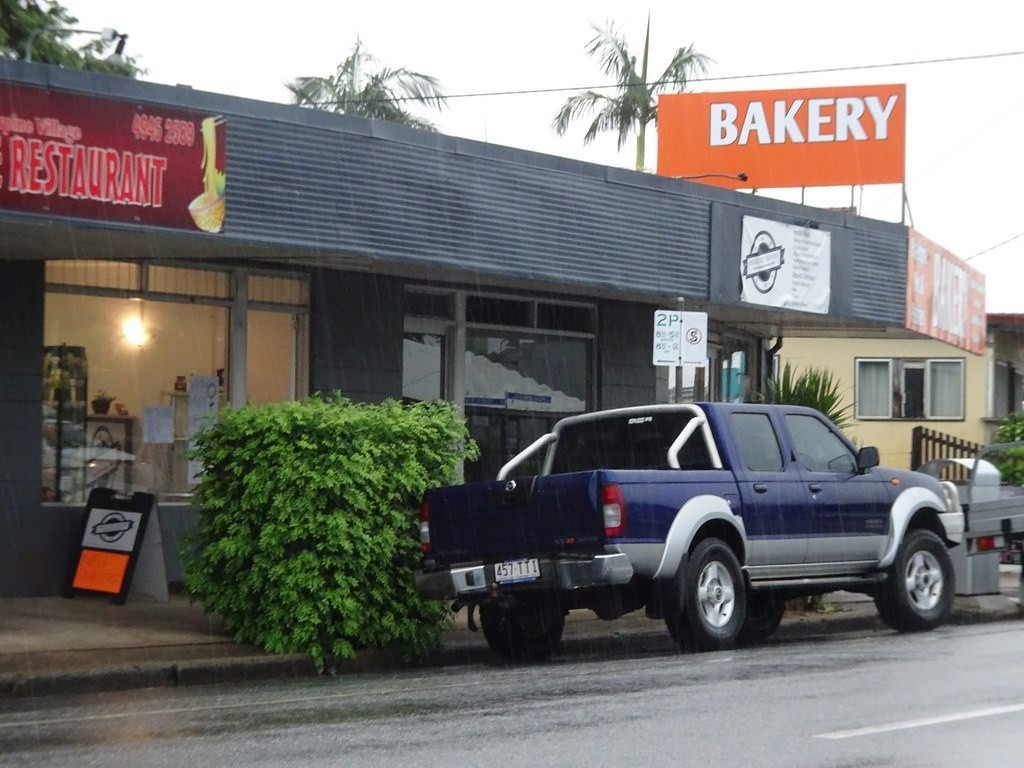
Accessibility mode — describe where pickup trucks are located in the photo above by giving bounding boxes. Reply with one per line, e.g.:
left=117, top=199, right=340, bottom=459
left=412, top=402, right=963, bottom=656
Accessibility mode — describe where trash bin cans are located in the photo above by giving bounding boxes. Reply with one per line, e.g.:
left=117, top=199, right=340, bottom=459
left=912, top=457, right=1000, bottom=598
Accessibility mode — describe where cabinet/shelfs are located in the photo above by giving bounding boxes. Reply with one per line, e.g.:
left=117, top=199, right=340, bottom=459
left=43, top=344, right=88, bottom=506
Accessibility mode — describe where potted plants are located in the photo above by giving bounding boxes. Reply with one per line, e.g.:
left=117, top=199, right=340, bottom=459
left=91, top=389, right=116, bottom=415
